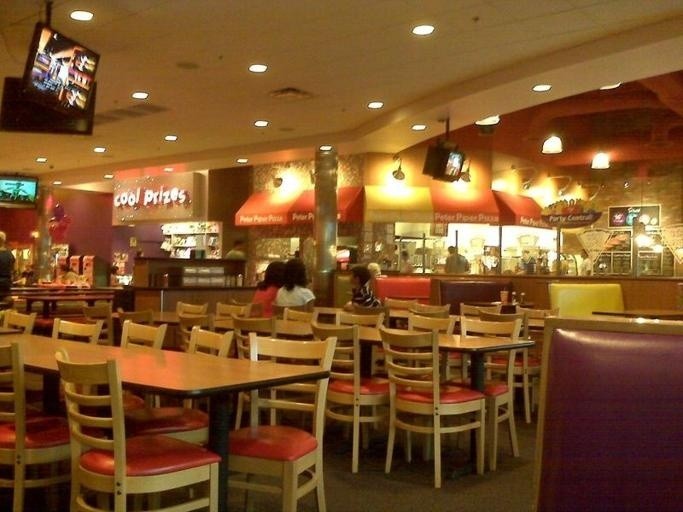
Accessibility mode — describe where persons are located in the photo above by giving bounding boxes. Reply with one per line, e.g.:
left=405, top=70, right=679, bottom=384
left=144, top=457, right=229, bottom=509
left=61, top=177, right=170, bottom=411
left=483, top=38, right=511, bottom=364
left=56, top=264, right=87, bottom=284
left=348, top=265, right=381, bottom=309
left=224, top=239, right=246, bottom=259
left=444, top=246, right=470, bottom=274
left=400, top=251, right=412, bottom=272
left=518, top=249, right=549, bottom=275
left=580, top=249, right=593, bottom=276
left=253, top=259, right=316, bottom=319
left=0, top=232, right=16, bottom=310
left=110, top=266, right=125, bottom=286
left=21, top=263, right=34, bottom=279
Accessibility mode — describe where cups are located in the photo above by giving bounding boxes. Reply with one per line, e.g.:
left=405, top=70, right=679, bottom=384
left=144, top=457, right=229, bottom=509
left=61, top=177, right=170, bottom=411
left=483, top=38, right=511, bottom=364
left=500, top=291, right=508, bottom=303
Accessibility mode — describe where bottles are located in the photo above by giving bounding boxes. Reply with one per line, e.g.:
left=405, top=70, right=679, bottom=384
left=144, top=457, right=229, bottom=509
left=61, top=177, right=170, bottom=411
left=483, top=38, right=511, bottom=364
left=520, top=292, right=526, bottom=304
left=511, top=292, right=517, bottom=303
left=542, top=199, right=592, bottom=215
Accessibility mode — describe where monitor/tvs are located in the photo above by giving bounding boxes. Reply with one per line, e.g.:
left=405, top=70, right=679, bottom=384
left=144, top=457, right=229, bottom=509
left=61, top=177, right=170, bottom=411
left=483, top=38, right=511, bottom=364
left=0, top=77, right=96, bottom=136
left=423, top=145, right=463, bottom=177
left=0, top=176, right=39, bottom=204
left=23, top=22, right=100, bottom=115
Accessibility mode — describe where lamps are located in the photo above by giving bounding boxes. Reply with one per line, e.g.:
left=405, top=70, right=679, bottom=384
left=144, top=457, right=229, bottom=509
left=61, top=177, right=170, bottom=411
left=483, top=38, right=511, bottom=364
left=634, top=185, right=651, bottom=249
left=475, top=116, right=501, bottom=134
left=393, top=157, right=405, bottom=182
left=269, top=172, right=284, bottom=189
left=461, top=157, right=475, bottom=183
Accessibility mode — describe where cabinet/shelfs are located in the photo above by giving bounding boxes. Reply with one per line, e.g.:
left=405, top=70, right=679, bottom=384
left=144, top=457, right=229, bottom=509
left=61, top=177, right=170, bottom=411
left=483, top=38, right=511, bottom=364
left=164, top=224, right=220, bottom=261
